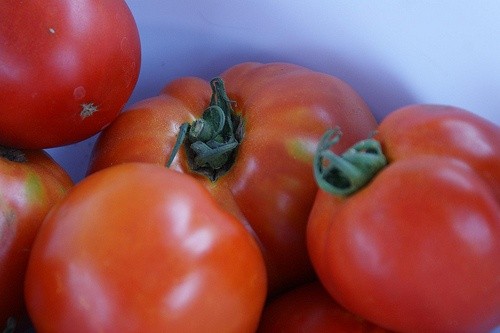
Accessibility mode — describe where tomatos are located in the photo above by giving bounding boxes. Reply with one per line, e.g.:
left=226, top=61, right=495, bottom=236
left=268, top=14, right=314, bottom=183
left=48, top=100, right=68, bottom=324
left=305, top=103, right=500, bottom=333
left=0, top=0, right=380, bottom=332
